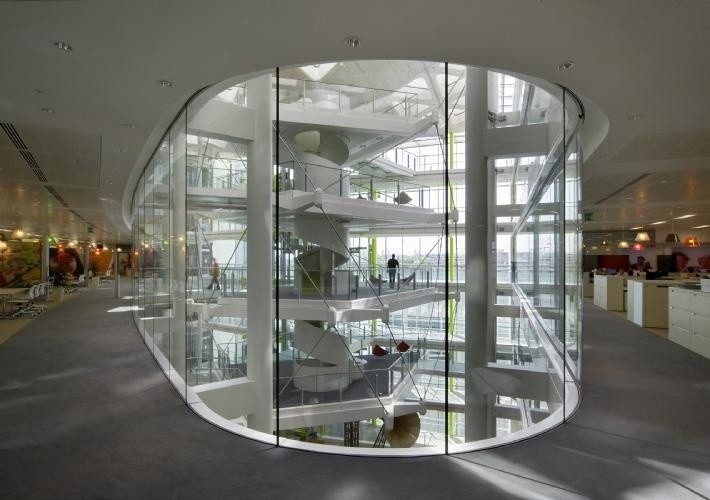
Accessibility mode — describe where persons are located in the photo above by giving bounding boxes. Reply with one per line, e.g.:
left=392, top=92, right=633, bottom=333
left=387, top=254, right=399, bottom=288
left=206, top=257, right=220, bottom=290
left=673, top=252, right=710, bottom=273
left=627, top=256, right=649, bottom=273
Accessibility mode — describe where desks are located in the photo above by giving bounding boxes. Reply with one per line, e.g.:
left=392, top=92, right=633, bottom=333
left=0, top=288, right=30, bottom=319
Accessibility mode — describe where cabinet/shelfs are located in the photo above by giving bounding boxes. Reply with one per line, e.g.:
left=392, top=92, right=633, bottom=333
left=667, top=287, right=710, bottom=359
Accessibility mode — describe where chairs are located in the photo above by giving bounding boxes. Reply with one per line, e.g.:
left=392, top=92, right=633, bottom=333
left=7, top=281, right=52, bottom=319
